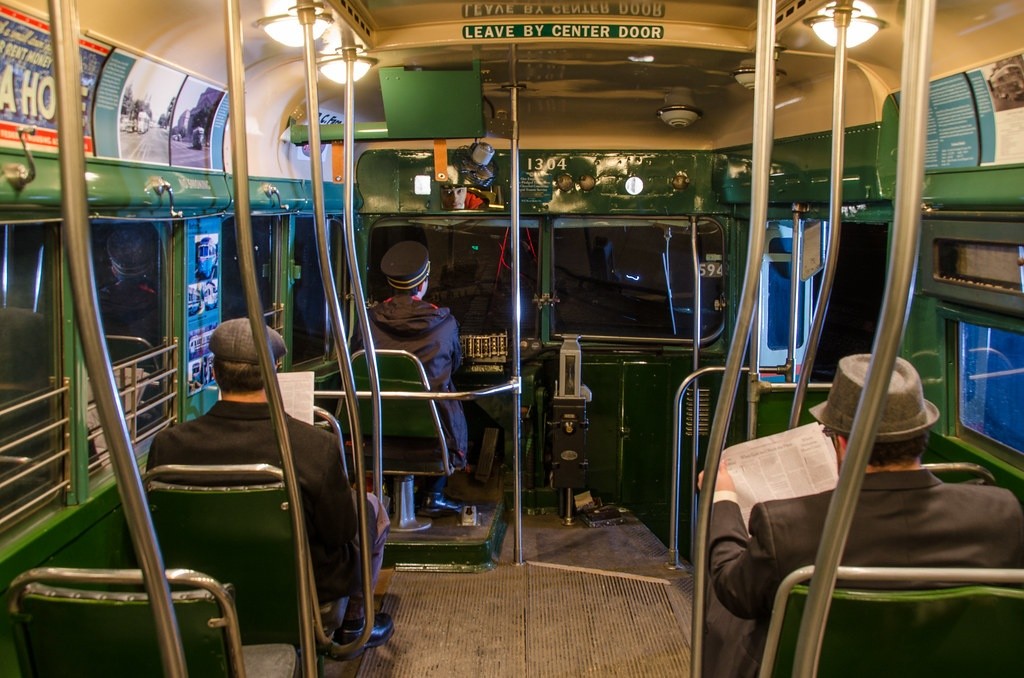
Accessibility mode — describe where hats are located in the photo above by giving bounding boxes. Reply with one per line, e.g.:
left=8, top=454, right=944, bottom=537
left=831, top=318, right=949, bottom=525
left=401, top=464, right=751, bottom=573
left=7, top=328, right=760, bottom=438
left=208, top=318, right=287, bottom=365
left=808, top=353, right=941, bottom=443
left=381, top=241, right=431, bottom=290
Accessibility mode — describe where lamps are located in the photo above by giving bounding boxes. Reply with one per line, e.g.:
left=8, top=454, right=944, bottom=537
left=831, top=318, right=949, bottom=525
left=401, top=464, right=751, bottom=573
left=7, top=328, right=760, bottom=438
left=656, top=106, right=706, bottom=127
left=257, top=13, right=333, bottom=47
left=802, top=15, right=887, bottom=50
left=730, top=67, right=786, bottom=91
left=311, top=53, right=378, bottom=87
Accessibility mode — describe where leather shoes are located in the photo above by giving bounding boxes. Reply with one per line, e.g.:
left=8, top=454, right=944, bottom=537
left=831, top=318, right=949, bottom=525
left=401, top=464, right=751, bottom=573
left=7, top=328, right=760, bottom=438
left=334, top=613, right=395, bottom=660
left=420, top=492, right=463, bottom=512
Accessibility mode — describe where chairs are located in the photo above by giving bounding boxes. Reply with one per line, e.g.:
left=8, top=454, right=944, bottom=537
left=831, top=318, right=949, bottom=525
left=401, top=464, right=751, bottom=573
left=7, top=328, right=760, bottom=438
left=140, top=464, right=349, bottom=678
left=334, top=347, right=458, bottom=532
left=5, top=566, right=302, bottom=678
left=758, top=566, right=1024, bottom=678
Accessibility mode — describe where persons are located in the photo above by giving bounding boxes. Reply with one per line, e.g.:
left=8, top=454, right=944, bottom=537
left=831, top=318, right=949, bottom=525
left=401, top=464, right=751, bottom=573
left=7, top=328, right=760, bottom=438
left=146, top=315, right=398, bottom=663
left=103, top=225, right=162, bottom=304
left=441, top=187, right=491, bottom=209
left=698, top=353, right=1024, bottom=677
left=349, top=241, right=468, bottom=512
left=0, top=307, right=47, bottom=501
